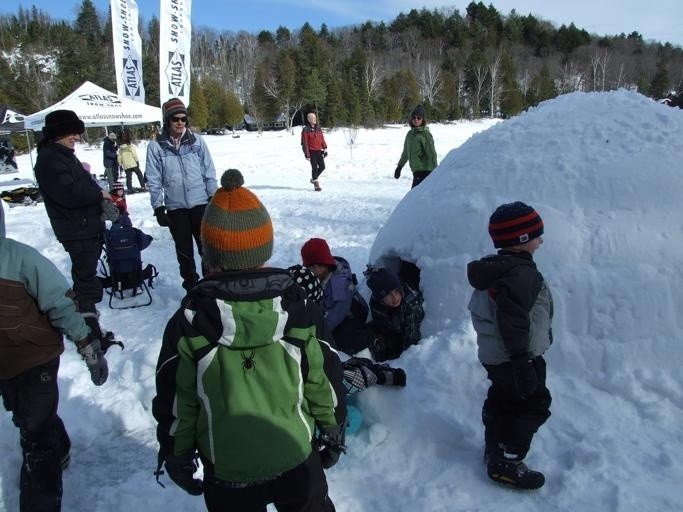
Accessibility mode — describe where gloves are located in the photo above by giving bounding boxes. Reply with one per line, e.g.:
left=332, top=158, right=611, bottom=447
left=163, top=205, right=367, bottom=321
left=394, top=166, right=402, bottom=179
left=164, top=456, right=201, bottom=496
left=77, top=339, right=108, bottom=386
left=153, top=206, right=170, bottom=227
left=508, top=354, right=539, bottom=396
left=317, top=424, right=344, bottom=469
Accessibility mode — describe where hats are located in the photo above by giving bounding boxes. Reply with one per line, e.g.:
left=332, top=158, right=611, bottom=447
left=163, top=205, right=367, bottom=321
left=411, top=104, right=426, bottom=119
left=161, top=98, right=188, bottom=116
left=487, top=202, right=546, bottom=249
left=112, top=181, right=124, bottom=189
left=287, top=265, right=322, bottom=301
left=368, top=269, right=403, bottom=296
left=200, top=169, right=274, bottom=270
left=301, top=238, right=336, bottom=268
left=42, top=110, right=85, bottom=137
left=109, top=131, right=131, bottom=143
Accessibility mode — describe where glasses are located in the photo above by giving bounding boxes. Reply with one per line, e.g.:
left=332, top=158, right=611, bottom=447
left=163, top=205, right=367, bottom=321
left=169, top=116, right=187, bottom=122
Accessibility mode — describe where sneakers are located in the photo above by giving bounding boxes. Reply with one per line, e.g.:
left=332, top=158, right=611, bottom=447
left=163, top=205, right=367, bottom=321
left=127, top=187, right=146, bottom=193
left=486, top=455, right=544, bottom=489
left=310, top=179, right=321, bottom=191
left=374, top=363, right=407, bottom=387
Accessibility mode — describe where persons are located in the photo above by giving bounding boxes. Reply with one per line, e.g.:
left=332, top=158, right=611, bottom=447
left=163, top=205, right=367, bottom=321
left=286, top=264, right=406, bottom=398
left=109, top=183, right=127, bottom=212
left=394, top=104, right=438, bottom=189
left=145, top=98, right=218, bottom=294
left=467, top=200, right=553, bottom=490
left=113, top=206, right=132, bottom=228
left=152, top=169, right=348, bottom=512
left=301, top=112, right=328, bottom=191
left=366, top=264, right=425, bottom=362
left=102, top=132, right=118, bottom=191
left=0, top=198, right=108, bottom=512
left=117, top=140, right=146, bottom=194
left=33, top=110, right=115, bottom=342
left=301, top=238, right=368, bottom=355
